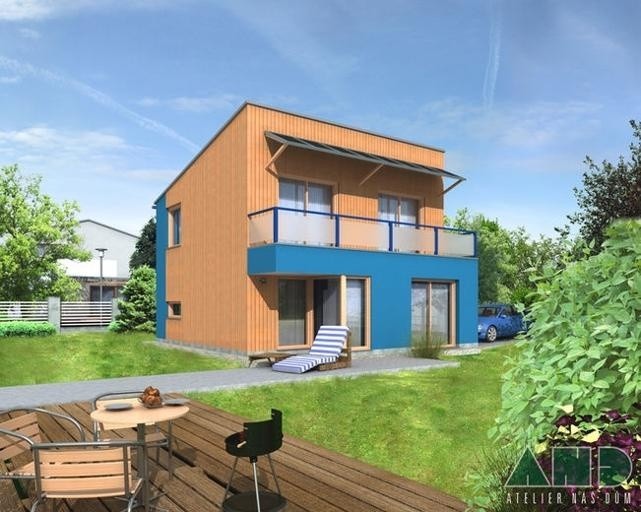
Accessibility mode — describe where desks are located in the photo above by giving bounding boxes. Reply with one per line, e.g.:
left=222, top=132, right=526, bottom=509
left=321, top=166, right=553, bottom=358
left=249, top=352, right=297, bottom=368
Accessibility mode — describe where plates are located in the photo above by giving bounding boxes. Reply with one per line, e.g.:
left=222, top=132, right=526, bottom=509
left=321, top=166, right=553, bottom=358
left=103, top=403, right=132, bottom=411
left=167, top=398, right=191, bottom=405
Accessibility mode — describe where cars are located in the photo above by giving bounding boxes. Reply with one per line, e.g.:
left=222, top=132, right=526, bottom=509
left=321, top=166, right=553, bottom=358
left=478, top=304, right=532, bottom=342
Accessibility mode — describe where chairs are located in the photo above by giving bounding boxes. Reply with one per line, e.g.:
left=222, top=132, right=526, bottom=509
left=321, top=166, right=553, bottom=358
left=0, top=391, right=168, bottom=512
left=272, top=325, right=352, bottom=374
left=222, top=409, right=284, bottom=512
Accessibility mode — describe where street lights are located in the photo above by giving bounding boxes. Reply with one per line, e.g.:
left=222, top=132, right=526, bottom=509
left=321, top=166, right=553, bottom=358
left=95, top=248, right=108, bottom=326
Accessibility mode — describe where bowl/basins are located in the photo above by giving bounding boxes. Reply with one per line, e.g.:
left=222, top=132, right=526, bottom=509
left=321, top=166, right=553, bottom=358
left=137, top=397, right=165, bottom=410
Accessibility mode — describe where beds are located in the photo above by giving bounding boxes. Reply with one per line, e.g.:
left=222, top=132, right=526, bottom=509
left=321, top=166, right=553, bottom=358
left=89, top=403, right=189, bottom=512
